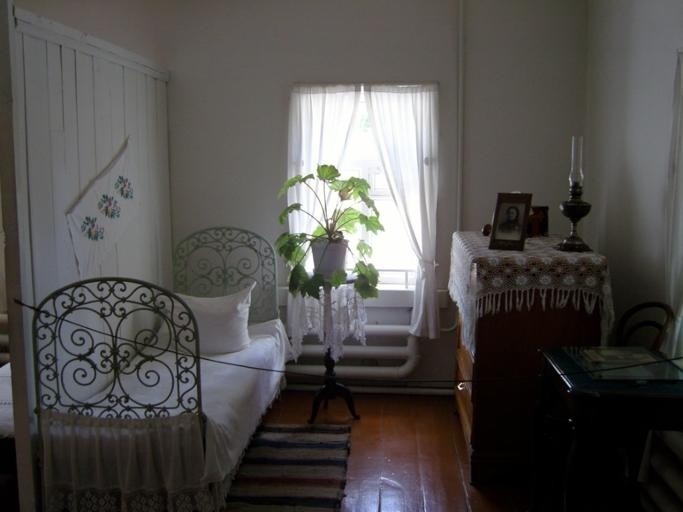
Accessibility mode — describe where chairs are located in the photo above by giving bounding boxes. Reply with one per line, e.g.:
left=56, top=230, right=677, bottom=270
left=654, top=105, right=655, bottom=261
left=609, top=301, right=674, bottom=478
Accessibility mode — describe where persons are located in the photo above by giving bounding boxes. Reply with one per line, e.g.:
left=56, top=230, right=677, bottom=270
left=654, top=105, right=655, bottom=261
left=498, top=206, right=521, bottom=236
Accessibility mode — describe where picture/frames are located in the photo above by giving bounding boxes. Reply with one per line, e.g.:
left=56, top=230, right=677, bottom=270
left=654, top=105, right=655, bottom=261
left=487, top=192, right=533, bottom=252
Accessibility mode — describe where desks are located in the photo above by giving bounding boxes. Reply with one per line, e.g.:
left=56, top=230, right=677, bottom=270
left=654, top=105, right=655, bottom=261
left=297, top=276, right=368, bottom=422
left=531, top=343, right=681, bottom=512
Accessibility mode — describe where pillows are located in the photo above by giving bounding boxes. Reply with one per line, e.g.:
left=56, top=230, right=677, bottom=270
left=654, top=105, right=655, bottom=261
left=154, top=279, right=260, bottom=355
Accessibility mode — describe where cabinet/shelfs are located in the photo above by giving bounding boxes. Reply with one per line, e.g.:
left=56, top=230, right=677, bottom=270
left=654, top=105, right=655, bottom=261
left=449, top=229, right=610, bottom=487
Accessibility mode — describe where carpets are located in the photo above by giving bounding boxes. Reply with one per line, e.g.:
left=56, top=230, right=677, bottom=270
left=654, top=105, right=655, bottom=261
left=225, top=419, right=352, bottom=512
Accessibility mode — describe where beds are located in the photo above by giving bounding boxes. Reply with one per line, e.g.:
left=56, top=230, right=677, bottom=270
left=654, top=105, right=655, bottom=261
left=28, top=226, right=291, bottom=512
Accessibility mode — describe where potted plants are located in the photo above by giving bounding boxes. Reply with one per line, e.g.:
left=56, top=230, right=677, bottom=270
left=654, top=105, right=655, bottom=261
left=274, top=163, right=383, bottom=297
